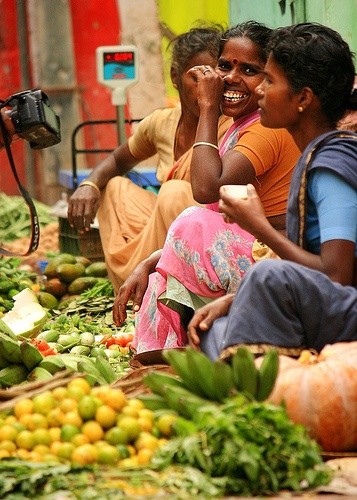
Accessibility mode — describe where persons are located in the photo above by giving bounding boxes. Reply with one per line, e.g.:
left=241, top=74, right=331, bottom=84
left=68, top=18, right=229, bottom=295
left=188, top=22, right=356, bottom=369
left=110, top=18, right=274, bottom=364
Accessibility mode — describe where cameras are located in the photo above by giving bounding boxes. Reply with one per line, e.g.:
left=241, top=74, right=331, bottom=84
left=4, top=87, right=62, bottom=151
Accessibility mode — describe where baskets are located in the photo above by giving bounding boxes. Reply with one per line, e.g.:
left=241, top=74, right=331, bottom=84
left=59, top=216, right=104, bottom=262
left=1, top=363, right=175, bottom=411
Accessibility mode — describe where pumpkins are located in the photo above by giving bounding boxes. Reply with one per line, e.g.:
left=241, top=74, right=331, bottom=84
left=253, top=340, right=357, bottom=452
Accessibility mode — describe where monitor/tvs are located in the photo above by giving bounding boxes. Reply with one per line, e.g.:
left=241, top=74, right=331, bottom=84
left=103, top=52, right=136, bottom=80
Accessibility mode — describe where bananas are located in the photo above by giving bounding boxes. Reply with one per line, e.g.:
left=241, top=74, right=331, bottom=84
left=0, top=319, right=124, bottom=387
left=136, top=345, right=280, bottom=440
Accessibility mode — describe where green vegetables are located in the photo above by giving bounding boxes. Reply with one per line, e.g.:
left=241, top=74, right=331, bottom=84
left=0, top=460, right=103, bottom=500
left=150, top=394, right=335, bottom=500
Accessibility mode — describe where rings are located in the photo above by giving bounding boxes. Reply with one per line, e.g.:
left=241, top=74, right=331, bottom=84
left=202, top=68, right=210, bottom=75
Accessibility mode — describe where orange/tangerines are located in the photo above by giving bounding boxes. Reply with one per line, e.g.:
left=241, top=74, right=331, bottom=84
left=0, top=378, right=178, bottom=474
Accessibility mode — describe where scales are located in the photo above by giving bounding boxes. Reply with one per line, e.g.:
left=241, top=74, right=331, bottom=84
left=94, top=45, right=140, bottom=145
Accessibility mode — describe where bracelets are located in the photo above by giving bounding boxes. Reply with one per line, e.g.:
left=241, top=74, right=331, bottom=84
left=192, top=141, right=220, bottom=151
left=77, top=181, right=101, bottom=195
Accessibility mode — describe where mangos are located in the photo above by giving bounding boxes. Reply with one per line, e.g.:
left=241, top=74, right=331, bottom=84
left=37, top=253, right=108, bottom=309
